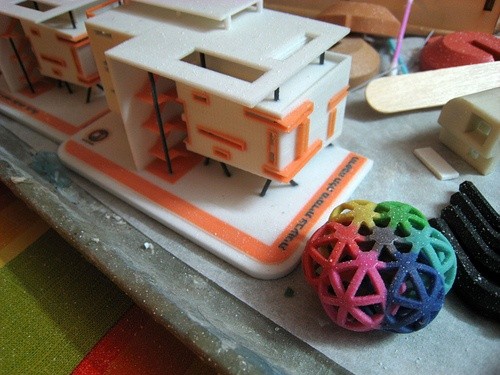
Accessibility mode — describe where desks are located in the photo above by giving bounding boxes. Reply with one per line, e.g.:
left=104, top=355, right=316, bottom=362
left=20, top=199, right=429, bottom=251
left=0, top=36, right=500, bottom=375
